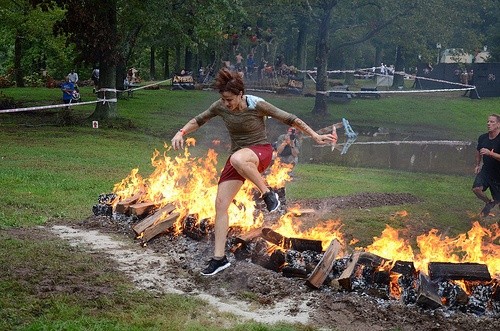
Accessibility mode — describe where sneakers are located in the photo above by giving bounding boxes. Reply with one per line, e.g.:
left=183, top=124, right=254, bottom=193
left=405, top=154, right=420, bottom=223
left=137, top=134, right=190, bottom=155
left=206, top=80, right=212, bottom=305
left=200, top=254, right=231, bottom=276
left=262, top=190, right=281, bottom=214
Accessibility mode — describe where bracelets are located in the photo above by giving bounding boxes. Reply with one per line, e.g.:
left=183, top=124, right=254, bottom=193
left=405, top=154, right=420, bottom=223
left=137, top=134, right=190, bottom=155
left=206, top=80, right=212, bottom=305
left=490, top=150, right=493, bottom=157
left=180, top=129, right=185, bottom=135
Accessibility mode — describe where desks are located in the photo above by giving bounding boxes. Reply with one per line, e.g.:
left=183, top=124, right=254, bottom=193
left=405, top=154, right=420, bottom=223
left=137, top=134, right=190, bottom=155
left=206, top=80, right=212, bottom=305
left=333, top=84, right=352, bottom=98
left=358, top=86, right=380, bottom=99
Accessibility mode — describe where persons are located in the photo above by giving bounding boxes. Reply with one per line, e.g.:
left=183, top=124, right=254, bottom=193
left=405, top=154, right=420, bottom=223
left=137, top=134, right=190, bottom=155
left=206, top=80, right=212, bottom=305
left=174, top=69, right=192, bottom=76
left=280, top=63, right=297, bottom=76
left=199, top=66, right=206, bottom=76
left=171, top=69, right=337, bottom=276
left=223, top=24, right=276, bottom=78
left=72, top=89, right=79, bottom=98
left=92, top=66, right=99, bottom=80
left=127, top=68, right=141, bottom=83
left=65, top=69, right=78, bottom=83
left=472, top=113, right=500, bottom=218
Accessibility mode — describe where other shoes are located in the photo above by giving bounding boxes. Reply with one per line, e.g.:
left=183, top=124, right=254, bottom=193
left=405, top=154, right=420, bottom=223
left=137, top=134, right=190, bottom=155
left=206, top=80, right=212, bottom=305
left=481, top=202, right=495, bottom=218
